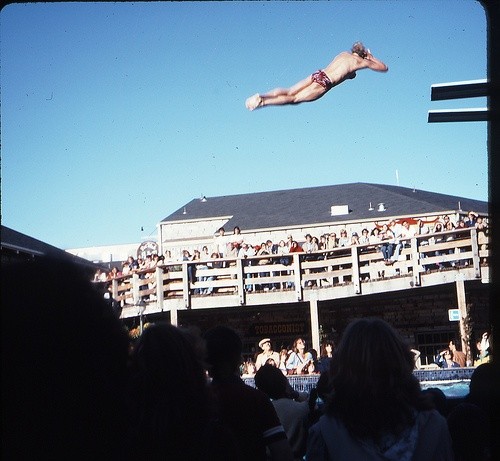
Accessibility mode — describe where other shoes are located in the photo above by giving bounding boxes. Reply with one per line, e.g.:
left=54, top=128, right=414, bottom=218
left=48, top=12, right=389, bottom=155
left=387, top=259, right=391, bottom=265
left=439, top=264, right=444, bottom=270
left=481, top=262, right=487, bottom=266
left=234, top=281, right=312, bottom=294
left=189, top=282, right=212, bottom=295
left=384, top=259, right=387, bottom=265
left=465, top=262, right=468, bottom=265
left=452, top=263, right=456, bottom=267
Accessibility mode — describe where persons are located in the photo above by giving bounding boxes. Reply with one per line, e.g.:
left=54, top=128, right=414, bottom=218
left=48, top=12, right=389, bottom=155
left=429, top=363, right=500, bottom=461
left=0, top=256, right=293, bottom=461
left=246, top=40, right=388, bottom=112
left=201, top=325, right=293, bottom=461
left=306, top=317, right=453, bottom=461
left=240, top=331, right=492, bottom=379
left=93, top=212, right=493, bottom=305
left=254, top=364, right=317, bottom=461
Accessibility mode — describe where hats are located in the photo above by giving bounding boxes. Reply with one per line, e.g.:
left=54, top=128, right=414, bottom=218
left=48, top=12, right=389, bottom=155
left=352, top=232, right=358, bottom=236
left=258, top=338, right=271, bottom=347
